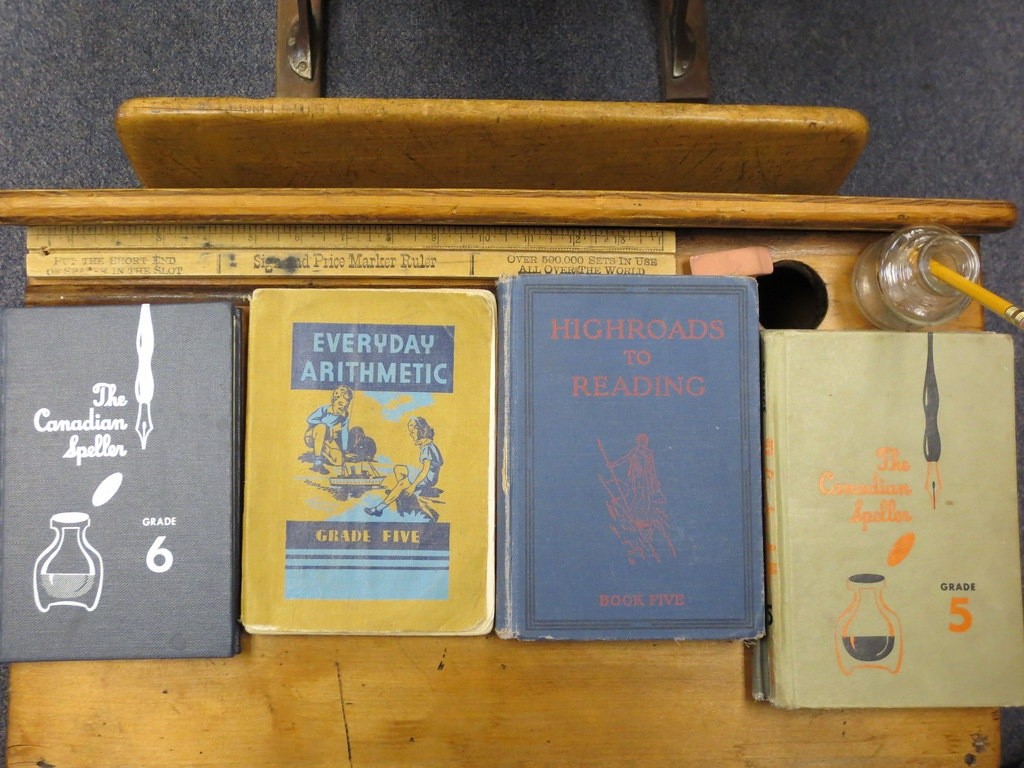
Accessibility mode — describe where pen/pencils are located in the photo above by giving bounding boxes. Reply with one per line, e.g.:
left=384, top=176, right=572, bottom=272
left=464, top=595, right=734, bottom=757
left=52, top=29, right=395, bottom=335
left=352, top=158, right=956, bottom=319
left=928, top=259, right=1024, bottom=338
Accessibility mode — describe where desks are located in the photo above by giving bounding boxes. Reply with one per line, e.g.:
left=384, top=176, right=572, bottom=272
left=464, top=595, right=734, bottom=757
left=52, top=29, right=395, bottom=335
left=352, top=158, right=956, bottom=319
left=0, top=190, right=1024, bottom=768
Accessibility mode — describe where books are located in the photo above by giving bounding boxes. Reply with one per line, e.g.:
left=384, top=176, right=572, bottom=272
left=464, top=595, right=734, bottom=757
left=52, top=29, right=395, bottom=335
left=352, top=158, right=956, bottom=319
left=0, top=299, right=243, bottom=666
left=490, top=269, right=767, bottom=644
left=752, top=324, right=1023, bottom=711
left=238, top=289, right=505, bottom=637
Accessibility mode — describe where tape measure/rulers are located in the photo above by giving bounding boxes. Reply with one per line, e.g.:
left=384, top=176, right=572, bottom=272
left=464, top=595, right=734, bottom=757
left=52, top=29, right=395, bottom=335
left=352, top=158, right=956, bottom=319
left=26, top=225, right=675, bottom=285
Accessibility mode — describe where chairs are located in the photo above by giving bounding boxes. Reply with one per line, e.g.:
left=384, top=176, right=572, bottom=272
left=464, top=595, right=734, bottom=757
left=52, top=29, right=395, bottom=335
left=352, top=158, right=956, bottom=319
left=117, top=96, right=871, bottom=192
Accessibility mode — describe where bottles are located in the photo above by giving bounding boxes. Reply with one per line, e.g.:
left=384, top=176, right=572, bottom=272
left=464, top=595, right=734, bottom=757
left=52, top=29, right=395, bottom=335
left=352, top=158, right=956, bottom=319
left=853, top=224, right=981, bottom=332
